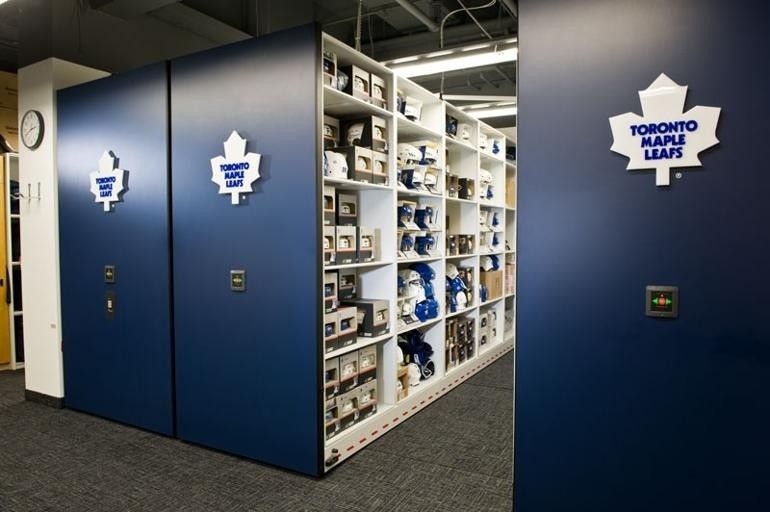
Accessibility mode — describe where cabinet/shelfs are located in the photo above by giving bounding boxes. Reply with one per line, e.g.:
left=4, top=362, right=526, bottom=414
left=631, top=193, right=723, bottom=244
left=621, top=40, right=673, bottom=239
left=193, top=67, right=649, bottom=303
left=512, top=0, right=769, bottom=512
left=0, top=20, right=516, bottom=482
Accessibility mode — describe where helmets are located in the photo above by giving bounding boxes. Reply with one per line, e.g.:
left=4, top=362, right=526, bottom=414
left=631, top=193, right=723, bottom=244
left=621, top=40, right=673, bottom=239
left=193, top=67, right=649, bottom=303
left=324, top=150, right=348, bottom=179
left=396, top=95, right=499, bottom=390
left=346, top=123, right=372, bottom=149
